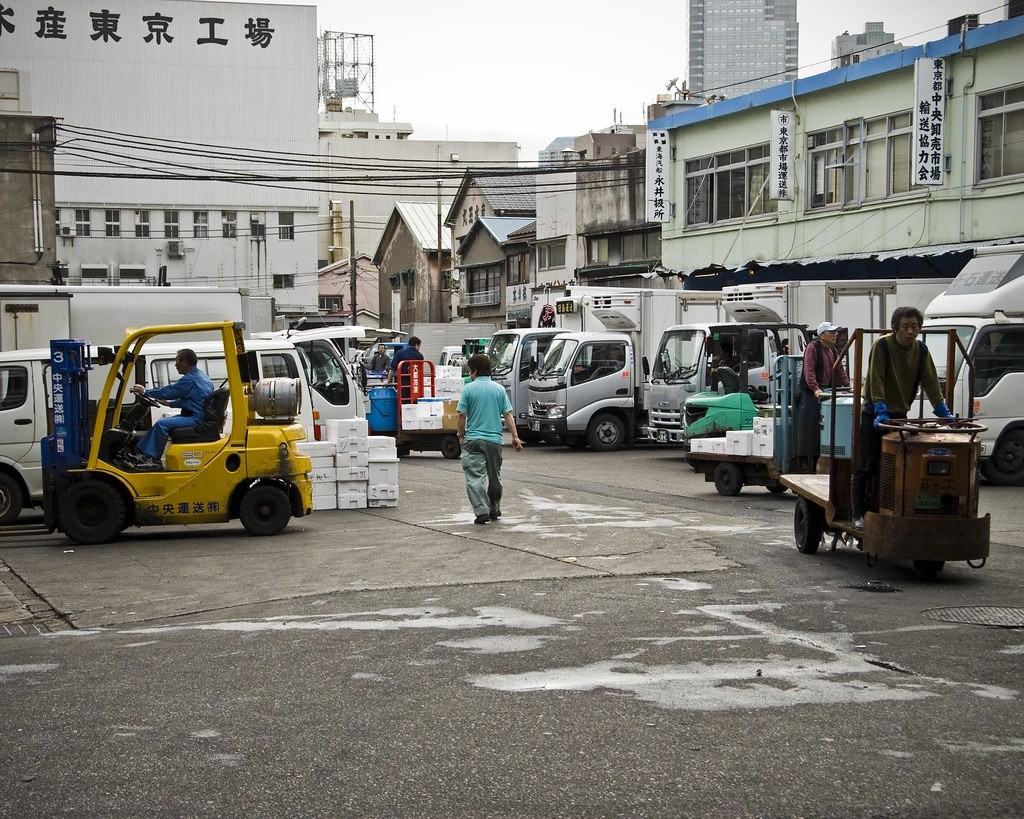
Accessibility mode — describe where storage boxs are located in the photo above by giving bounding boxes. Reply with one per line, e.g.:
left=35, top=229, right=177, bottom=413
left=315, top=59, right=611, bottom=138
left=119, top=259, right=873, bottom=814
left=691, top=416, right=793, bottom=457
left=402, top=365, right=464, bottom=429
left=295, top=418, right=400, bottom=513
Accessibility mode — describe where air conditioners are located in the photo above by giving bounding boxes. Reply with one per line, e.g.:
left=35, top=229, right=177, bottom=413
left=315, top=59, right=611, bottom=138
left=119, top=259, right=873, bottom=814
left=167, top=240, right=183, bottom=256
left=60, top=224, right=76, bottom=239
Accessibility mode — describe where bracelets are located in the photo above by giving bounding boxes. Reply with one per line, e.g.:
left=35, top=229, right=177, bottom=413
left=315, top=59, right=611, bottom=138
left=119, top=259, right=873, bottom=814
left=457, top=433, right=466, bottom=437
left=143, top=389, right=147, bottom=397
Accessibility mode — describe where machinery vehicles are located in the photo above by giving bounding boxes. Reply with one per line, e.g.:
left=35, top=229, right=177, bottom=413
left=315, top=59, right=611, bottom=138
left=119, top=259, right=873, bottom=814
left=780, top=324, right=990, bottom=574
left=680, top=323, right=866, bottom=496
left=462, top=318, right=516, bottom=387
left=365, top=358, right=463, bottom=459
left=0, top=319, right=314, bottom=544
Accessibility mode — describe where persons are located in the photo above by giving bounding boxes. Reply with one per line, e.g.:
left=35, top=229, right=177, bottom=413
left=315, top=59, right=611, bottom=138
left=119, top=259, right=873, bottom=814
left=456, top=355, right=523, bottom=523
left=851, top=307, right=955, bottom=531
left=363, top=344, right=389, bottom=374
left=127, top=349, right=214, bottom=471
left=789, top=322, right=853, bottom=476
left=382, top=336, right=424, bottom=404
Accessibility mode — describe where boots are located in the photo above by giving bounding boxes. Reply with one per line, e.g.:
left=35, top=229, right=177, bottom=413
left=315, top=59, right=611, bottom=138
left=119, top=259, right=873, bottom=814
left=851, top=477, right=869, bottom=530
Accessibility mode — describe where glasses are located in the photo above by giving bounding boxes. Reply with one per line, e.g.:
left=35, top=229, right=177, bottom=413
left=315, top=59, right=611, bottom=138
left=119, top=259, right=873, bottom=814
left=824, top=331, right=838, bottom=334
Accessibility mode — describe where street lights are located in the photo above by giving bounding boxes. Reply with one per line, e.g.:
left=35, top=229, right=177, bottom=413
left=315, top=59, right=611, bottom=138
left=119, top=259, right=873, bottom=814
left=328, top=246, right=357, bottom=325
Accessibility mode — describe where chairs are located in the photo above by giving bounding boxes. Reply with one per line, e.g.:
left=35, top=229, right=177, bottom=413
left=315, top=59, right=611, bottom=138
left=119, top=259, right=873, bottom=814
left=169, top=387, right=230, bottom=443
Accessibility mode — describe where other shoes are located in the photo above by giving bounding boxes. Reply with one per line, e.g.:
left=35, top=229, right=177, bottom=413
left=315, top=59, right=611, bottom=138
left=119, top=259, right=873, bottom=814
left=125, top=453, right=146, bottom=465
left=489, top=511, right=501, bottom=519
left=474, top=514, right=490, bottom=523
left=134, top=460, right=163, bottom=471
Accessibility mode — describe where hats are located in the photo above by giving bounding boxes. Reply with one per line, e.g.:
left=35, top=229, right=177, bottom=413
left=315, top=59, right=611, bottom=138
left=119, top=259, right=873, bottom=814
left=817, top=322, right=841, bottom=336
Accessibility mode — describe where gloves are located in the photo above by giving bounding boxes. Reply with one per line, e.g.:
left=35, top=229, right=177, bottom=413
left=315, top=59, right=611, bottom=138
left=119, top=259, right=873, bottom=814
left=932, top=403, right=956, bottom=423
left=873, top=400, right=889, bottom=430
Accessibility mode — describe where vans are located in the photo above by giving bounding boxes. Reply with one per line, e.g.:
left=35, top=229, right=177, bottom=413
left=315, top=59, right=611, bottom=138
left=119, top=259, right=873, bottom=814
left=0, top=340, right=321, bottom=527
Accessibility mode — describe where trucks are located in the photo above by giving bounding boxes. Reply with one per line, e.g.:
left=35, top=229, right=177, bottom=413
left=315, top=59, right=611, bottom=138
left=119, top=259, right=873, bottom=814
left=360, top=321, right=497, bottom=372
left=903, top=243, right=1024, bottom=486
left=488, top=286, right=726, bottom=452
left=640, top=279, right=960, bottom=446
left=0, top=284, right=366, bottom=440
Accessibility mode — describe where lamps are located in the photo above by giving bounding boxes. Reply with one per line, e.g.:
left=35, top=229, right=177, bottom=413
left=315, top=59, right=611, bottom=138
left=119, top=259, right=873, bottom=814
left=665, top=77, right=689, bottom=97
left=704, top=94, right=726, bottom=104
